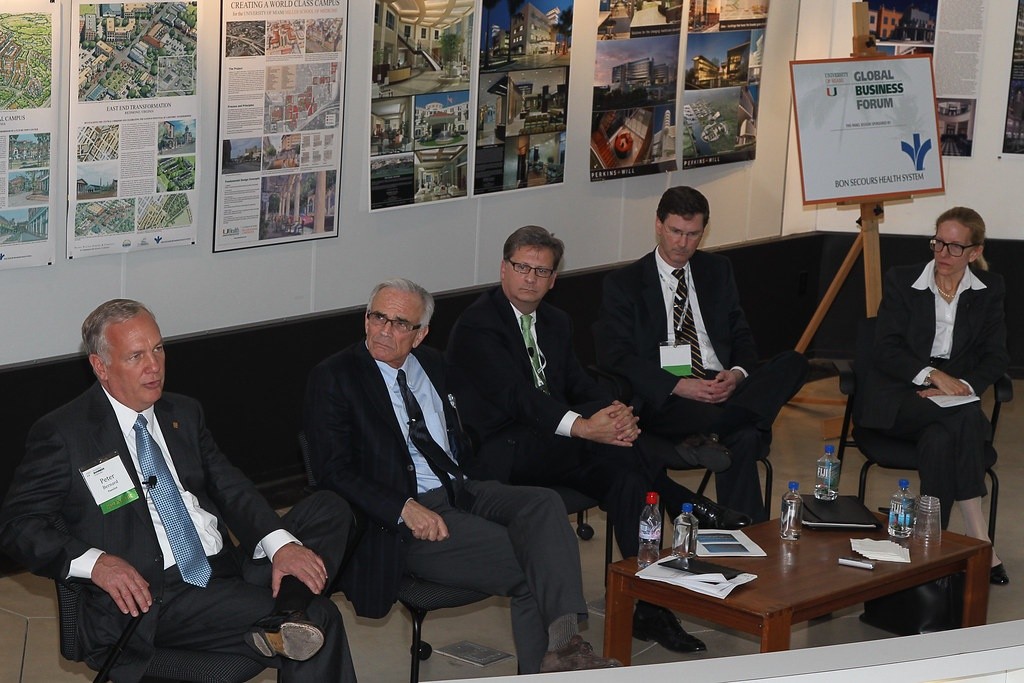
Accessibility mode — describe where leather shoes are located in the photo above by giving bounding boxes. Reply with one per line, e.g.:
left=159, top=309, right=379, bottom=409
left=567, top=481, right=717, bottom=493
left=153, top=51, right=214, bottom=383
left=675, top=433, right=733, bottom=474
left=691, top=493, right=753, bottom=529
left=243, top=611, right=324, bottom=662
left=990, top=562, right=1009, bottom=585
left=632, top=606, right=706, bottom=652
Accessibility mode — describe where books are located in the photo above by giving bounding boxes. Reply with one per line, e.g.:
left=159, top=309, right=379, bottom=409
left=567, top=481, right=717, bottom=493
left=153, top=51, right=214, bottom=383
left=799, top=493, right=884, bottom=533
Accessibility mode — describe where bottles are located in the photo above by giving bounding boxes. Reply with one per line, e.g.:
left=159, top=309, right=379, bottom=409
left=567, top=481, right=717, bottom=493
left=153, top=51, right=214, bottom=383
left=887, top=479, right=916, bottom=538
left=779, top=481, right=803, bottom=541
left=814, top=445, right=840, bottom=500
left=672, top=503, right=698, bottom=559
left=636, top=491, right=661, bottom=568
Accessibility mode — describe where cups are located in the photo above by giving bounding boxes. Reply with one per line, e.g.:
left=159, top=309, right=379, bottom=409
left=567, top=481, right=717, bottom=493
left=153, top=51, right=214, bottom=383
left=913, top=495, right=941, bottom=546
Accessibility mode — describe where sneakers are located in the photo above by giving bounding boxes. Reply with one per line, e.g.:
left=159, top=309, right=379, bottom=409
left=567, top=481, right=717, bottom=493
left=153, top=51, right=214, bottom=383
left=540, top=635, right=624, bottom=674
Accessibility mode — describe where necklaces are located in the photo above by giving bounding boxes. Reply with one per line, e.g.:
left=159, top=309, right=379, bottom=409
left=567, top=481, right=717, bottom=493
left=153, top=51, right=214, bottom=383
left=936, top=283, right=955, bottom=298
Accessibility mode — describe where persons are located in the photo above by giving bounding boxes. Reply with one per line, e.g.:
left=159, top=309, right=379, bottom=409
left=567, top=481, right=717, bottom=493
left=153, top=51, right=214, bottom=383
left=0, top=299, right=362, bottom=683
left=445, top=227, right=753, bottom=652
left=592, top=186, right=810, bottom=527
left=850, top=207, right=1010, bottom=587
left=302, top=278, right=626, bottom=675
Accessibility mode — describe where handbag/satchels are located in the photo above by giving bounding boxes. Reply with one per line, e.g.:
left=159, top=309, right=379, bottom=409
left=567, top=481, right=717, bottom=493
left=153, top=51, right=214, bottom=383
left=859, top=576, right=962, bottom=635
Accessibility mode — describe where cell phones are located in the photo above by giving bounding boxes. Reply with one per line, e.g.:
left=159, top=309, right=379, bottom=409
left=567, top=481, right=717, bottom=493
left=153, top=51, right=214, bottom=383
left=839, top=557, right=876, bottom=569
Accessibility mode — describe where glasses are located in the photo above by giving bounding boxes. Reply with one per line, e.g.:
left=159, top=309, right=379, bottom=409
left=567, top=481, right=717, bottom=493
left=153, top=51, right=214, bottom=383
left=663, top=225, right=702, bottom=240
left=367, top=310, right=421, bottom=331
left=509, top=260, right=554, bottom=278
left=929, top=236, right=975, bottom=257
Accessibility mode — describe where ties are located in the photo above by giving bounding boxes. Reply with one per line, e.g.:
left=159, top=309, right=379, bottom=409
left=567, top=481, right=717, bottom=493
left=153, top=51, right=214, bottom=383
left=397, top=370, right=464, bottom=507
left=520, top=315, right=550, bottom=395
left=133, top=414, right=213, bottom=588
left=672, top=268, right=705, bottom=380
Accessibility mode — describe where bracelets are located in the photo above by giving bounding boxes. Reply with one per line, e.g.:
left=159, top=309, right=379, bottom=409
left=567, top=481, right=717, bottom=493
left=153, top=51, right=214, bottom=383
left=925, top=369, right=936, bottom=385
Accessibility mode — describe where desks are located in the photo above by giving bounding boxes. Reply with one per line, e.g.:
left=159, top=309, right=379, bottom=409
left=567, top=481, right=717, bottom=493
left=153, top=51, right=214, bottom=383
left=603, top=512, right=992, bottom=666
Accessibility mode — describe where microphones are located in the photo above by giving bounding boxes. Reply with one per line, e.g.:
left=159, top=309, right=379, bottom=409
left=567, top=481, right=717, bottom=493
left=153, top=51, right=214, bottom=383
left=148, top=475, right=157, bottom=490
left=679, top=298, right=686, bottom=306
left=528, top=347, right=534, bottom=358
left=414, top=412, right=423, bottom=422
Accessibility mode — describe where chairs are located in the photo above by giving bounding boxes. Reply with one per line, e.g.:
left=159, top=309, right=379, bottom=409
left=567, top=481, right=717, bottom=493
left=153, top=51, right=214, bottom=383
left=54, top=577, right=267, bottom=683
left=833, top=316, right=1013, bottom=547
left=294, top=431, right=492, bottom=683
left=551, top=362, right=772, bottom=603
